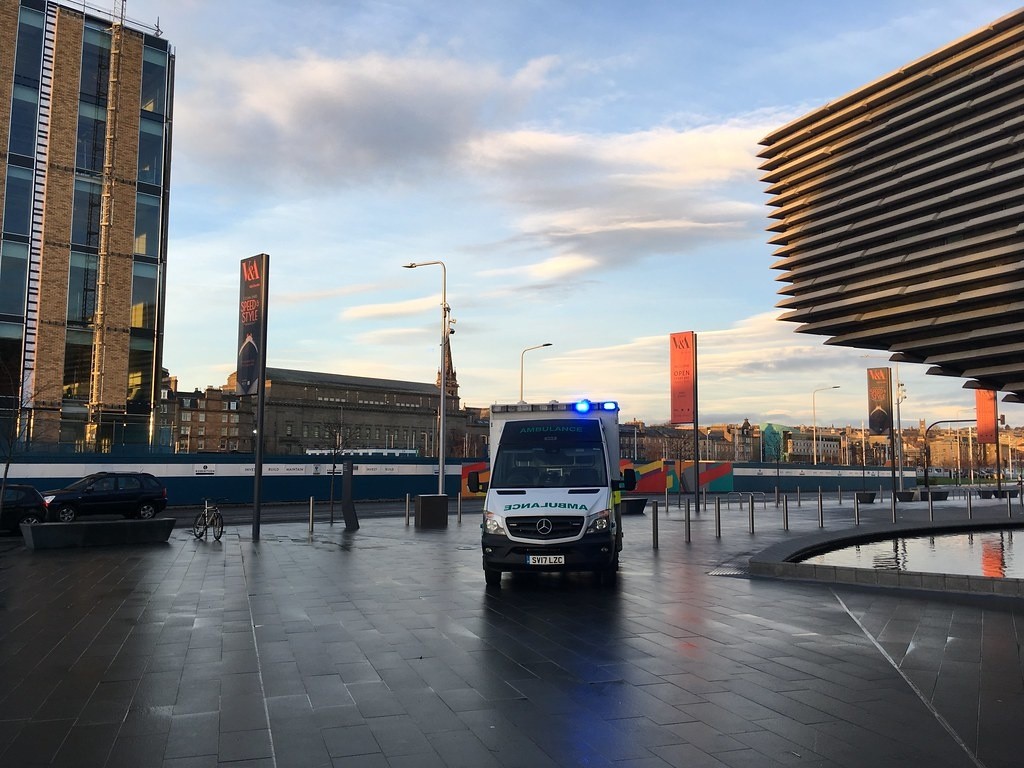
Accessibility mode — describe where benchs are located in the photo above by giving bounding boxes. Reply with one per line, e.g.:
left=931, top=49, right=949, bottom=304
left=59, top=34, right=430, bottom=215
left=978, top=490, right=1019, bottom=499
left=621, top=498, right=648, bottom=514
left=921, top=492, right=949, bottom=501
left=20, top=518, right=176, bottom=551
left=856, top=493, right=876, bottom=503
left=896, top=492, right=915, bottom=501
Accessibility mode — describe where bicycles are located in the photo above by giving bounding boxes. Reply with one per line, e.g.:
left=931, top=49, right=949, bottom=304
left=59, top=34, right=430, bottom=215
left=193, top=498, right=229, bottom=540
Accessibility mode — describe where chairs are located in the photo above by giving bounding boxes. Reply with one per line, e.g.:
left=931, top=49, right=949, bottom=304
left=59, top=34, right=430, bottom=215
left=569, top=469, right=600, bottom=486
left=507, top=475, right=533, bottom=485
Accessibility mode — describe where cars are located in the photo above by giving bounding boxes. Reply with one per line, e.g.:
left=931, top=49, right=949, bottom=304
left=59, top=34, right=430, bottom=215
left=0, top=484, right=49, bottom=536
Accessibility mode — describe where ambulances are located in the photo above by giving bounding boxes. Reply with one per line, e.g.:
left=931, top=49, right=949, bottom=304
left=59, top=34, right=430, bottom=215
left=481, top=400, right=624, bottom=585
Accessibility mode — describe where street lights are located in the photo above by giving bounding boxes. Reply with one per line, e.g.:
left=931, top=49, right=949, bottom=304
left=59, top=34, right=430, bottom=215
left=894, top=382, right=907, bottom=492
left=813, top=386, right=840, bottom=466
left=957, top=408, right=976, bottom=485
left=402, top=262, right=446, bottom=495
left=519, top=343, right=553, bottom=404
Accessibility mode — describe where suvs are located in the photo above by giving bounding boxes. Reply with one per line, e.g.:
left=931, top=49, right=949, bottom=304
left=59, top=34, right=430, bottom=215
left=40, top=472, right=168, bottom=522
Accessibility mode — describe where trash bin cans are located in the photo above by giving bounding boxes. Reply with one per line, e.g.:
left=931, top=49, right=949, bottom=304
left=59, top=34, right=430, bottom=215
left=414, top=494, right=448, bottom=529
